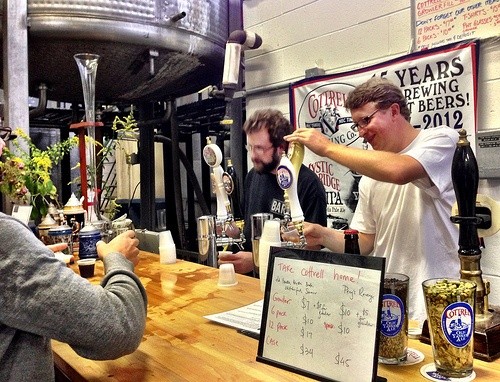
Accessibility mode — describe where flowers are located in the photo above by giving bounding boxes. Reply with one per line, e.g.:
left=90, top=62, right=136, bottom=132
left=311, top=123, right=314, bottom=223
left=0, top=104, right=141, bottom=219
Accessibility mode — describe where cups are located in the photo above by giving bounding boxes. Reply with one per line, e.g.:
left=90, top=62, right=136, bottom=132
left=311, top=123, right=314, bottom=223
left=421, top=277, right=477, bottom=378
left=219, top=264, right=238, bottom=286
left=104, top=228, right=130, bottom=276
left=77, top=258, right=97, bottom=278
left=157, top=209, right=168, bottom=230
left=251, top=212, right=301, bottom=294
left=218, top=251, right=233, bottom=264
left=377, top=273, right=410, bottom=362
left=159, top=229, right=177, bottom=263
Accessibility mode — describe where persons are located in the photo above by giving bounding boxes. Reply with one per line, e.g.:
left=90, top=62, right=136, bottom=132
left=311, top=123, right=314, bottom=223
left=0, top=212, right=148, bottom=382
left=212, top=109, right=327, bottom=278
left=282, top=78, right=463, bottom=335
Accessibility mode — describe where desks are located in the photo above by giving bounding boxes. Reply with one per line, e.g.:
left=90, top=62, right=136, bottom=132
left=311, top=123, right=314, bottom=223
left=49, top=247, right=500, bottom=382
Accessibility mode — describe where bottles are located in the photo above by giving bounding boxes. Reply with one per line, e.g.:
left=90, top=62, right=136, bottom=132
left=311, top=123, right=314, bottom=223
left=37, top=192, right=103, bottom=260
left=343, top=230, right=361, bottom=255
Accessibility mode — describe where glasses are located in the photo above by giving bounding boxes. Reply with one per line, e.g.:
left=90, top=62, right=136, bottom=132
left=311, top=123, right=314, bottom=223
left=351, top=104, right=388, bottom=132
left=245, top=143, right=276, bottom=155
